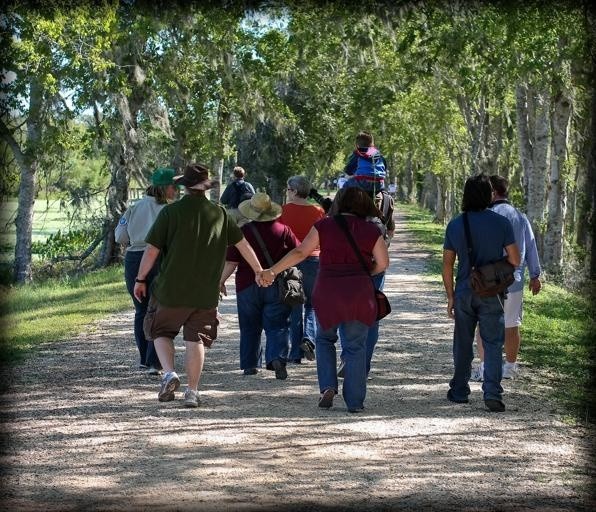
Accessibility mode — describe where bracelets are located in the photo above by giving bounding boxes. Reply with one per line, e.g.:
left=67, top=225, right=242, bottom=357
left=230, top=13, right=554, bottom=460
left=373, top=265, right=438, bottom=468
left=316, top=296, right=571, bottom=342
left=269, top=268, right=277, bottom=279
left=134, top=277, right=146, bottom=284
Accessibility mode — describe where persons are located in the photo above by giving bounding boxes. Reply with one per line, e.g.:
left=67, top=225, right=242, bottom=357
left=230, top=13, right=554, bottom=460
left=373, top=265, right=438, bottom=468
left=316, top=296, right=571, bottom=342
left=217, top=190, right=303, bottom=380
left=470, top=174, right=543, bottom=385
left=219, top=166, right=254, bottom=227
left=326, top=176, right=332, bottom=196
left=438, top=173, right=519, bottom=413
left=337, top=173, right=347, bottom=189
left=131, top=163, right=276, bottom=409
left=276, top=175, right=325, bottom=367
left=332, top=177, right=338, bottom=190
left=307, top=190, right=397, bottom=383
left=342, top=130, right=388, bottom=194
left=259, top=184, right=391, bottom=413
left=113, top=166, right=181, bottom=377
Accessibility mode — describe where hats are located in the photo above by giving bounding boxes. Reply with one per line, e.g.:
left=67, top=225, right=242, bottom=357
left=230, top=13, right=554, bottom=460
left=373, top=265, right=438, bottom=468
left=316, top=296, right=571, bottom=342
left=150, top=168, right=177, bottom=186
left=238, top=192, right=283, bottom=222
left=172, top=163, right=220, bottom=191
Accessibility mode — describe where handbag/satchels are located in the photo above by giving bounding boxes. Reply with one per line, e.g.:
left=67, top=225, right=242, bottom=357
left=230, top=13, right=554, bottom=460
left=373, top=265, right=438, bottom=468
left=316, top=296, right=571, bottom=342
left=276, top=266, right=308, bottom=306
left=466, top=256, right=516, bottom=298
left=375, top=288, right=392, bottom=321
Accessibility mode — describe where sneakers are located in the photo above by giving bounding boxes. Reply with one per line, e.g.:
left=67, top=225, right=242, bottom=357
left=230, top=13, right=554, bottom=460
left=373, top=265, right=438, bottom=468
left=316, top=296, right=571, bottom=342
left=484, top=398, right=506, bottom=412
left=271, top=359, right=288, bottom=380
left=158, top=369, right=181, bottom=402
left=183, top=386, right=201, bottom=408
left=318, top=388, right=335, bottom=408
left=447, top=389, right=469, bottom=403
left=139, top=354, right=163, bottom=375
left=349, top=405, right=365, bottom=412
left=286, top=339, right=316, bottom=363
left=502, top=363, right=520, bottom=380
left=243, top=366, right=259, bottom=375
left=469, top=364, right=485, bottom=382
left=336, top=359, right=346, bottom=378
